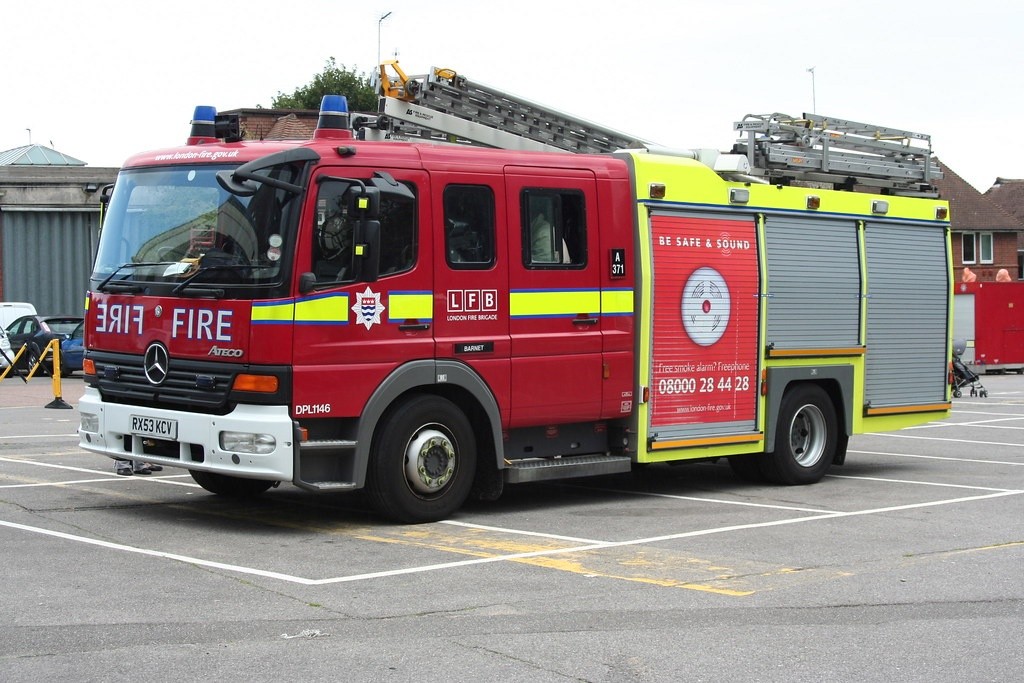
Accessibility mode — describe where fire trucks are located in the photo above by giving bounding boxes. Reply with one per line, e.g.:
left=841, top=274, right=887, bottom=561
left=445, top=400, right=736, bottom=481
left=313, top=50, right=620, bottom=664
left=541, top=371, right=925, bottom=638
left=79, top=54, right=956, bottom=522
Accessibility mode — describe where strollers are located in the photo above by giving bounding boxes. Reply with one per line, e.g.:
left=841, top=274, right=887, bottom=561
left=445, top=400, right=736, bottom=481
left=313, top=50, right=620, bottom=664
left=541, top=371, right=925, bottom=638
left=949, top=340, right=989, bottom=399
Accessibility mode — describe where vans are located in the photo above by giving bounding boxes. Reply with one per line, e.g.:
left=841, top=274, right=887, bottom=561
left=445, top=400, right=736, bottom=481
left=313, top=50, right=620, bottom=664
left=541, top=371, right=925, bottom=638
left=0, top=301, right=38, bottom=333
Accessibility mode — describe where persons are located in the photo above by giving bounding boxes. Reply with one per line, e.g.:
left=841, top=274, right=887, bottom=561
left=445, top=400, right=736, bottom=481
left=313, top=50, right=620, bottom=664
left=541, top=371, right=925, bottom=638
left=113, top=460, right=152, bottom=478
left=529, top=207, right=572, bottom=262
left=141, top=462, right=166, bottom=472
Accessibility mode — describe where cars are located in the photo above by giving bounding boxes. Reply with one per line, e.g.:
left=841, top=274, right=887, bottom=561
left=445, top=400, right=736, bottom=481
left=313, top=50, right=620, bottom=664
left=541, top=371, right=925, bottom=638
left=57, top=318, right=86, bottom=378
left=0, top=326, right=15, bottom=373
left=6, top=315, right=83, bottom=378
